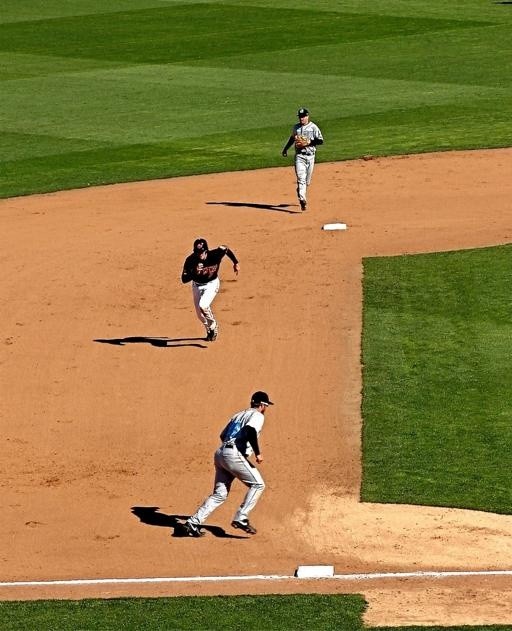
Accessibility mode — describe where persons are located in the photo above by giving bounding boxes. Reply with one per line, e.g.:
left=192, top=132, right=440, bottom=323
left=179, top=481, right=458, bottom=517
left=182, top=238, right=240, bottom=342
left=179, top=391, right=275, bottom=538
left=282, top=110, right=324, bottom=211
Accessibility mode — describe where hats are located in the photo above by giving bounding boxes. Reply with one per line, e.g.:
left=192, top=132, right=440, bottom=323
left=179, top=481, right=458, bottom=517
left=251, top=390, right=275, bottom=406
left=296, top=108, right=309, bottom=118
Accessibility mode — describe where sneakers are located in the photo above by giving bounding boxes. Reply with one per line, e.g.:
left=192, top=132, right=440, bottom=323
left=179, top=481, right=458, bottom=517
left=299, top=200, right=307, bottom=211
left=230, top=518, right=257, bottom=535
left=206, top=326, right=219, bottom=341
left=183, top=521, right=206, bottom=537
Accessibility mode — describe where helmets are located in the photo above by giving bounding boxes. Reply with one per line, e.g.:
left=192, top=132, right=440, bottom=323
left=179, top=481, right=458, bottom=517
left=193, top=238, right=208, bottom=254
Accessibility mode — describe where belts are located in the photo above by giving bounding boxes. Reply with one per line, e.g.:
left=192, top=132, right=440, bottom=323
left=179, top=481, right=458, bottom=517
left=222, top=445, right=235, bottom=449
left=298, top=152, right=314, bottom=156
